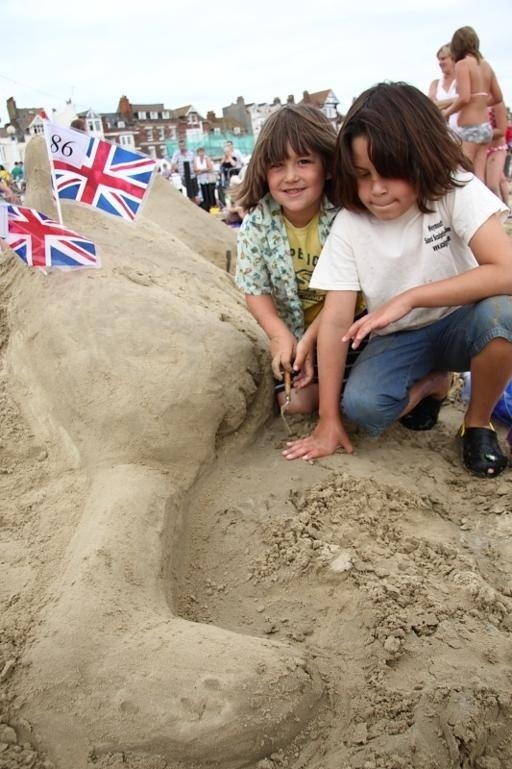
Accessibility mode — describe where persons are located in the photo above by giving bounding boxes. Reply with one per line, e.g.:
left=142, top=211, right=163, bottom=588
left=281, top=82, right=512, bottom=478
left=429, top=27, right=511, bottom=208
left=0, top=161, right=26, bottom=207
left=162, top=140, right=245, bottom=225
left=222, top=103, right=370, bottom=416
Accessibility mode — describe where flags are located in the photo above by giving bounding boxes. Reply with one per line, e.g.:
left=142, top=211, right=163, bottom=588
left=0, top=202, right=101, bottom=269
left=44, top=121, right=159, bottom=225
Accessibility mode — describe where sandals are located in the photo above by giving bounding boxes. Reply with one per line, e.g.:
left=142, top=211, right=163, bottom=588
left=458, top=415, right=507, bottom=478
left=398, top=388, right=448, bottom=431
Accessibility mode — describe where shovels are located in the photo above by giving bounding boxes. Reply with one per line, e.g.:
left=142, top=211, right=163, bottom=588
left=281, top=364, right=292, bottom=435
